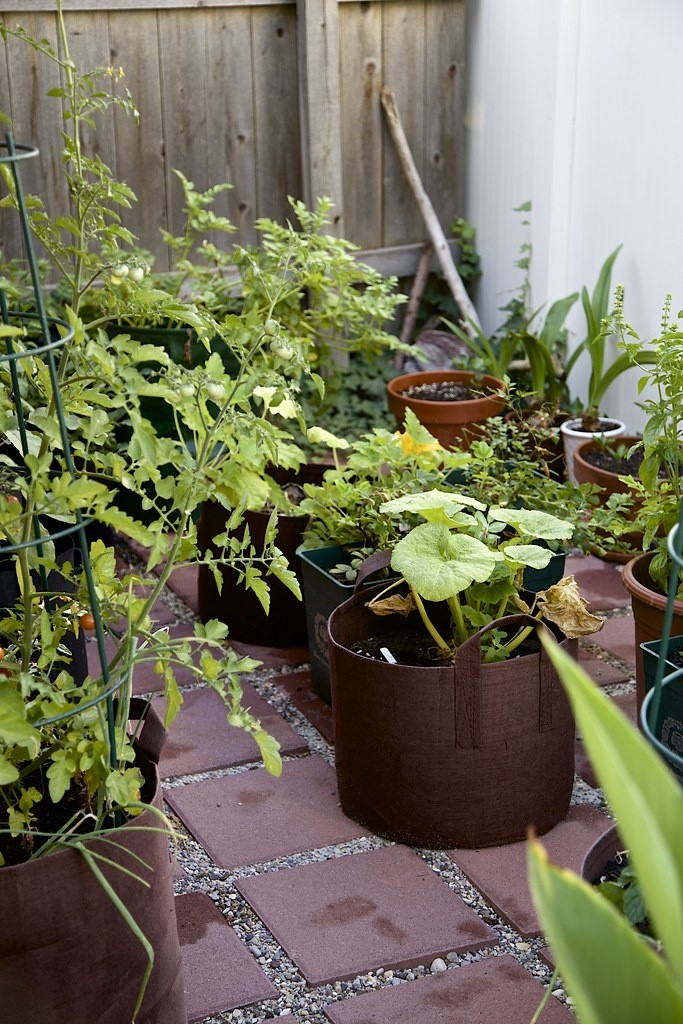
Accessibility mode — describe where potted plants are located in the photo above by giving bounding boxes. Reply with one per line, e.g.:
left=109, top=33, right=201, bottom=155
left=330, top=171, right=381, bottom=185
left=0, top=0, right=683, bottom=1024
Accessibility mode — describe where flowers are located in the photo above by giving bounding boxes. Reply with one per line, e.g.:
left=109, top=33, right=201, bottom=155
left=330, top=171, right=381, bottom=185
left=302, top=407, right=647, bottom=588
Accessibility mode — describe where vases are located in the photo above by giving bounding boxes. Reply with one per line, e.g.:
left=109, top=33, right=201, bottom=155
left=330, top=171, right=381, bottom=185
left=295, top=538, right=419, bottom=707
